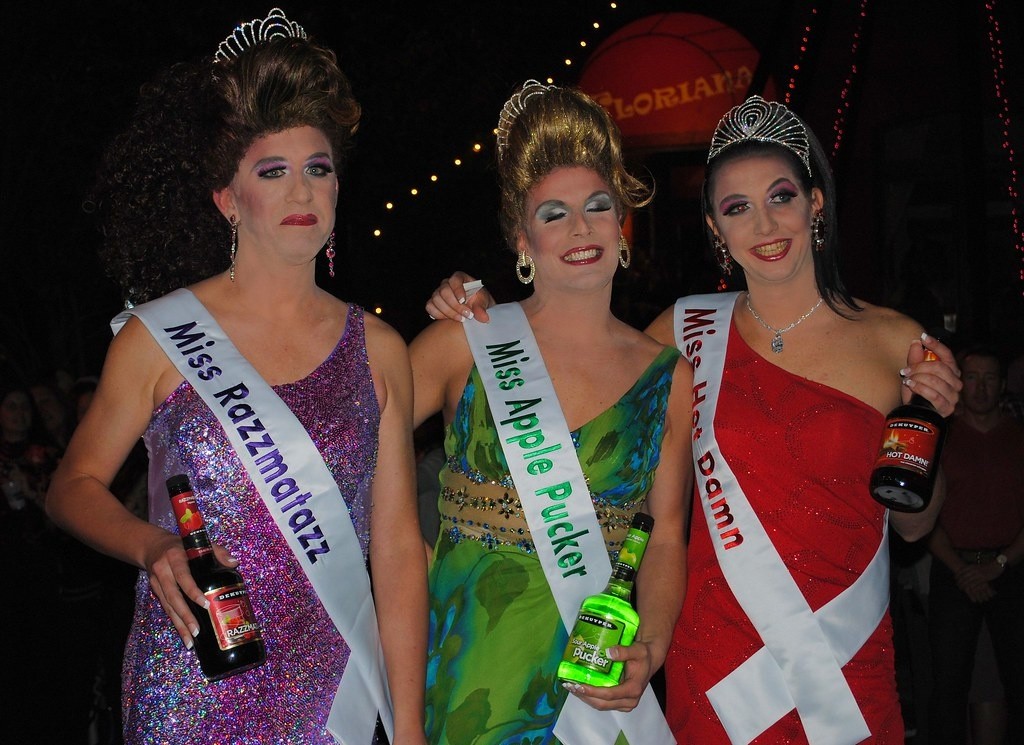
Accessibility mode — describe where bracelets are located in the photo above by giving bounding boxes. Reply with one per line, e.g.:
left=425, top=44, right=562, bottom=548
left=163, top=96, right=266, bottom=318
left=995, top=552, right=1008, bottom=568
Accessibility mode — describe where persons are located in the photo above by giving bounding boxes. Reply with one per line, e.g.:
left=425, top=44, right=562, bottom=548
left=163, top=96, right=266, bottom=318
left=0, top=368, right=146, bottom=744
left=406, top=91, right=697, bottom=744
left=890, top=346, right=1024, bottom=744
left=421, top=96, right=962, bottom=744
left=42, top=8, right=430, bottom=745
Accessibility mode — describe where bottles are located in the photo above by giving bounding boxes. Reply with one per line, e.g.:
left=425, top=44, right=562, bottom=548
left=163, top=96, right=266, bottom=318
left=166, top=474, right=267, bottom=682
left=1, top=462, right=27, bottom=511
left=556, top=512, right=655, bottom=688
left=869, top=335, right=950, bottom=512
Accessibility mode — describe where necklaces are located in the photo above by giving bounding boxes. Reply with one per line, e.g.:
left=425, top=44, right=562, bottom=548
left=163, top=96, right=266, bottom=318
left=746, top=295, right=826, bottom=353
